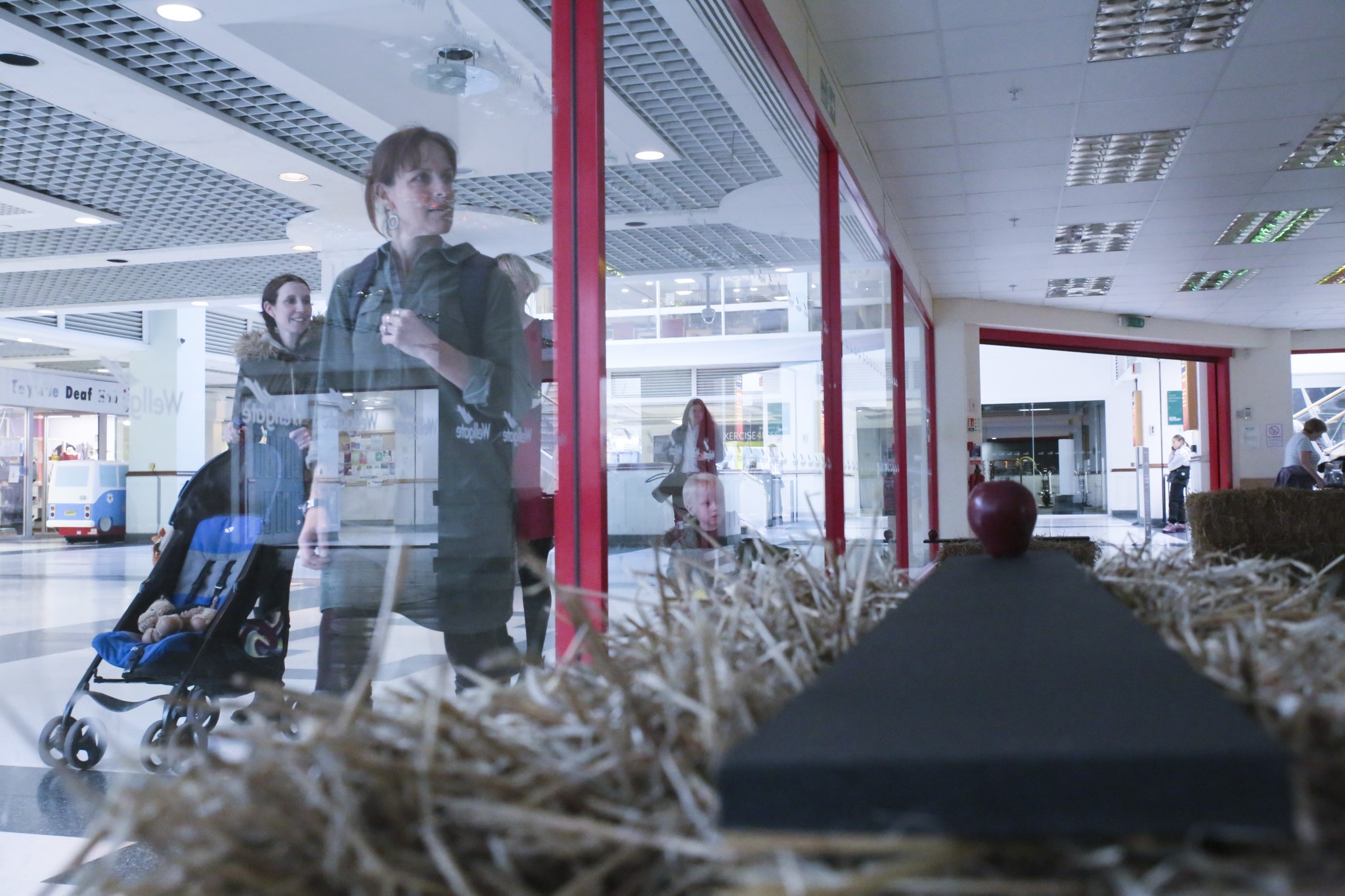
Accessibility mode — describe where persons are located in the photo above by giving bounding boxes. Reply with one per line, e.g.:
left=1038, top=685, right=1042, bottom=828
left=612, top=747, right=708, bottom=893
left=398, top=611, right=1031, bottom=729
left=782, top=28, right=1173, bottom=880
left=664, top=399, right=724, bottom=530
left=492, top=254, right=553, bottom=686
left=1318, top=455, right=1345, bottom=474
left=297, top=127, right=534, bottom=710
left=665, top=472, right=799, bottom=600
left=225, top=272, right=329, bottom=721
left=1274, top=417, right=1326, bottom=492
left=1161, top=434, right=1191, bottom=533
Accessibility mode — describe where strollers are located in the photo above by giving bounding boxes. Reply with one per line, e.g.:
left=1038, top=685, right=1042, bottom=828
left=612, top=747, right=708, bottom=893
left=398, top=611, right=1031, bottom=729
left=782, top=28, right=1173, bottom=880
left=37, top=421, right=310, bottom=779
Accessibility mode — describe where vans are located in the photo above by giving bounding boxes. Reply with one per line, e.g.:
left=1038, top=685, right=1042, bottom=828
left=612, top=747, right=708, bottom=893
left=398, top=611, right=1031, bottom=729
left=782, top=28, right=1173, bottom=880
left=45, top=461, right=128, bottom=543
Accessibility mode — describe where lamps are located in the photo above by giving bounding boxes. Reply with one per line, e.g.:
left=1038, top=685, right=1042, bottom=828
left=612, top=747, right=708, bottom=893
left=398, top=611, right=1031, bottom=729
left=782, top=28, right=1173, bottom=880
left=408, top=44, right=501, bottom=98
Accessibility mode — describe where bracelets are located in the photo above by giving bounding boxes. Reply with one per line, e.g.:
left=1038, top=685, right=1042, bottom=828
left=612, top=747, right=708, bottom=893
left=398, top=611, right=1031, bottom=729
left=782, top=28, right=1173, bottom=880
left=301, top=498, right=323, bottom=513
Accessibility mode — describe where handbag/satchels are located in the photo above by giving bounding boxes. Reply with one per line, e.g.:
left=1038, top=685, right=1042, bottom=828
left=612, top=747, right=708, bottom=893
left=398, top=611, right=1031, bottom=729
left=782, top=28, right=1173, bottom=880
left=645, top=471, right=675, bottom=503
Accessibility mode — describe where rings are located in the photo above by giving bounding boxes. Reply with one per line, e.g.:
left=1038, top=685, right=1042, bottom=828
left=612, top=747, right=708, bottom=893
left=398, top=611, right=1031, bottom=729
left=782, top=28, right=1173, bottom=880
left=383, top=325, right=388, bottom=335
left=385, top=315, right=391, bottom=324
left=394, top=308, right=401, bottom=316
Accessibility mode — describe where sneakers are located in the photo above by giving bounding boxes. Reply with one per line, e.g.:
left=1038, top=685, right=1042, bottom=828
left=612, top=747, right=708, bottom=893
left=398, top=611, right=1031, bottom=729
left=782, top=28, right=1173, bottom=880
left=1161, top=523, right=1176, bottom=533
left=1173, top=522, right=1187, bottom=532
